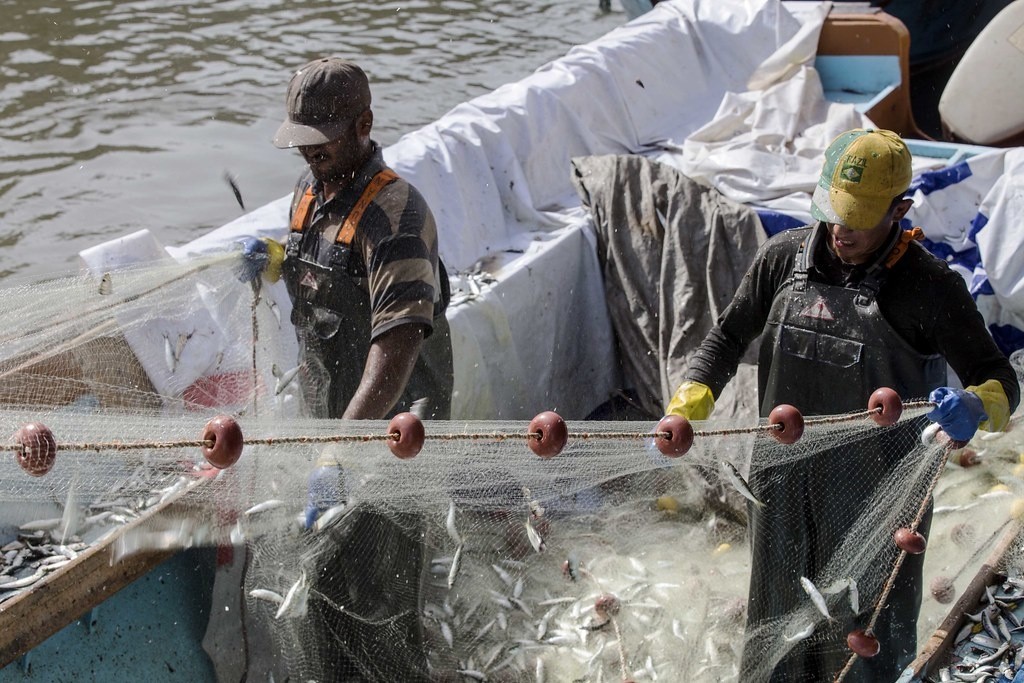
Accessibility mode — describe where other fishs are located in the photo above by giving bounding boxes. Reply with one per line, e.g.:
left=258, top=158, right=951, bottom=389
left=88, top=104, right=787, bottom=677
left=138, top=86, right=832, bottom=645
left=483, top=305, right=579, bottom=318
left=0, top=261, right=1023, bottom=683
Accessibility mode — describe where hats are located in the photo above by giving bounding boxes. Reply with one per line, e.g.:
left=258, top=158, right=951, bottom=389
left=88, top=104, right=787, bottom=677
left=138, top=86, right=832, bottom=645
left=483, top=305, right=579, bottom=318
left=273, top=57, right=371, bottom=149
left=811, top=128, right=913, bottom=230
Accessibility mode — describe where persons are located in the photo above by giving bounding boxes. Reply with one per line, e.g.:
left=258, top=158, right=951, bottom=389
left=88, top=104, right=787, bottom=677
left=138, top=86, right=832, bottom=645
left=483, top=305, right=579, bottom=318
left=232, top=56, right=456, bottom=681
left=662, top=126, right=1022, bottom=680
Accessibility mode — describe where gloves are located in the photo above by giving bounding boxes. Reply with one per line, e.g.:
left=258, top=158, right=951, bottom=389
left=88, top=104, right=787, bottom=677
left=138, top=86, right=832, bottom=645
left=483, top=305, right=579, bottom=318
left=228, top=236, right=285, bottom=284
left=667, top=380, right=715, bottom=420
left=926, top=380, right=1010, bottom=441
left=305, top=458, right=347, bottom=529
